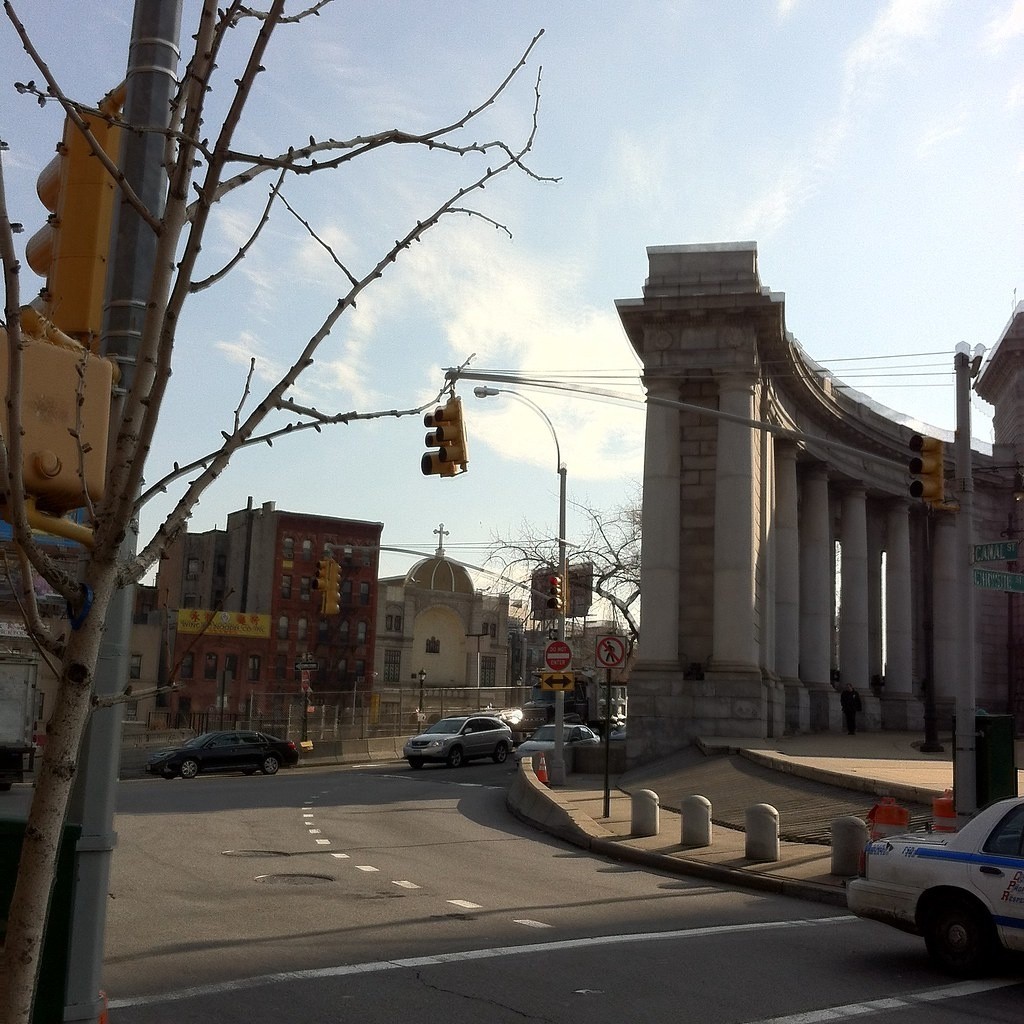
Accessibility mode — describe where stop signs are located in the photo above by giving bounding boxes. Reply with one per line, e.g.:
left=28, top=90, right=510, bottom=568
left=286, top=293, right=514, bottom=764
left=545, top=641, right=572, bottom=672
left=300, top=671, right=310, bottom=693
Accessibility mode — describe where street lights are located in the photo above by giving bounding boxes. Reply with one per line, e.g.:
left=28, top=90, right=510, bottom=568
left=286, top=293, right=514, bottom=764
left=417, top=668, right=426, bottom=734
left=474, top=385, right=567, bottom=786
left=465, top=633, right=493, bottom=710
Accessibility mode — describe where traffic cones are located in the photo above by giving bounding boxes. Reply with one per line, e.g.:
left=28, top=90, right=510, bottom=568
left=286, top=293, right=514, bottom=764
left=538, top=753, right=550, bottom=783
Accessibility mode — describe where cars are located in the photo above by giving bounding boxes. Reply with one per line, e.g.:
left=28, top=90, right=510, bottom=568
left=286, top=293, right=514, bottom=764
left=513, top=724, right=601, bottom=767
left=847, top=795, right=1024, bottom=979
left=145, top=730, right=298, bottom=779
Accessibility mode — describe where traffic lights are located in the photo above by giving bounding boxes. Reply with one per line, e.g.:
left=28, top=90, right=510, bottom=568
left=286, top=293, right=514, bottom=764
left=547, top=577, right=563, bottom=610
left=19, top=103, right=121, bottom=355
left=909, top=435, right=945, bottom=502
left=421, top=397, right=469, bottom=477
left=312, top=560, right=342, bottom=614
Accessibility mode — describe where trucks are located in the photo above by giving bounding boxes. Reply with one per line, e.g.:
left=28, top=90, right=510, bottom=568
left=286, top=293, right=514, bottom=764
left=500, top=667, right=628, bottom=748
left=0, top=652, right=41, bottom=791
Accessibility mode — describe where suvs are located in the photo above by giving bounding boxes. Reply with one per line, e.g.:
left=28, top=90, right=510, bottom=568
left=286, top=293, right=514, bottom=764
left=403, top=714, right=513, bottom=769
